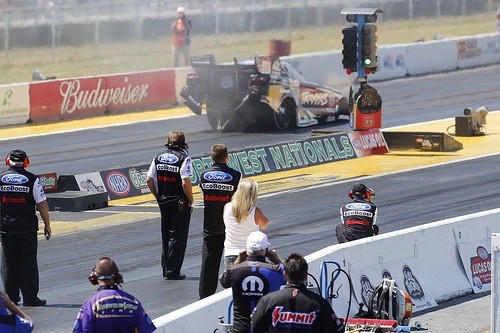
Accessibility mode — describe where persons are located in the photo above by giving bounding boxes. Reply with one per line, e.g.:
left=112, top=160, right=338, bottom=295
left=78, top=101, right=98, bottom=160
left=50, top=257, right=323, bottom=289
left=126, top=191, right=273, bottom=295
left=0, top=290, right=34, bottom=333
left=171, top=7, right=192, bottom=67
left=220, top=231, right=287, bottom=333
left=0, top=149, right=51, bottom=306
left=335, top=184, right=379, bottom=244
left=71, top=257, right=156, bottom=333
left=250, top=254, right=344, bottom=333
left=223, top=179, right=269, bottom=270
left=198, top=144, right=242, bottom=300
left=147, top=131, right=193, bottom=280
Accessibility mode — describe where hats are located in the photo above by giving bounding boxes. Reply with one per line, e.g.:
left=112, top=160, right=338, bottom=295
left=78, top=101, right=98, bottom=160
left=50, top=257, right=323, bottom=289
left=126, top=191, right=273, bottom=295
left=178, top=7, right=185, bottom=13
left=10, top=149, right=27, bottom=163
left=246, top=231, right=271, bottom=251
left=352, top=183, right=367, bottom=196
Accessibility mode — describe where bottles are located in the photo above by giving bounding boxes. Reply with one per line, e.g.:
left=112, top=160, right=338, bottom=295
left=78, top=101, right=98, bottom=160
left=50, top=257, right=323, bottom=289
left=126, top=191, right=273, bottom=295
left=414, top=321, right=422, bottom=329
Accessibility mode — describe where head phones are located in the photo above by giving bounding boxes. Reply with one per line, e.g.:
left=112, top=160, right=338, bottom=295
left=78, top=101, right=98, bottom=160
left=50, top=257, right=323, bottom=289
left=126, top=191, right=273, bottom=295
left=87, top=271, right=123, bottom=285
left=164, top=143, right=189, bottom=150
left=5, top=150, right=30, bottom=167
left=348, top=187, right=371, bottom=200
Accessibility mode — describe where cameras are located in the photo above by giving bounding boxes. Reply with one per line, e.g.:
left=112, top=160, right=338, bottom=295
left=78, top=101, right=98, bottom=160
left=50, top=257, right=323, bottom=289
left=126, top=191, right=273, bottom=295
left=180, top=15, right=185, bottom=19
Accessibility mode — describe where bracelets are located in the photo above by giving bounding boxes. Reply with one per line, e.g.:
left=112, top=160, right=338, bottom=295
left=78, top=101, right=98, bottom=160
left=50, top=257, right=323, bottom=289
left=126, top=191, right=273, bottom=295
left=189, top=200, right=194, bottom=204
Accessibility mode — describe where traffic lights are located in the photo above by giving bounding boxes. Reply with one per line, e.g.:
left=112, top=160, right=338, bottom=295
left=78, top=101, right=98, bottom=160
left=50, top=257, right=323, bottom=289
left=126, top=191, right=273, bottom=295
left=363, top=23, right=377, bottom=68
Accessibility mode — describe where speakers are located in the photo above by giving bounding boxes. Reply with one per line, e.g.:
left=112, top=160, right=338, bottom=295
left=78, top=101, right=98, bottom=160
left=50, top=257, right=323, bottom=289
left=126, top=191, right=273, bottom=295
left=455, top=116, right=475, bottom=136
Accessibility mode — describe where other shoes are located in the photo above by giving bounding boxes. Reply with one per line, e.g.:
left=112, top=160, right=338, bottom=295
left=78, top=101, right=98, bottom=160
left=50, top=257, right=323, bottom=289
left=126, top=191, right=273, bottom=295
left=14, top=302, right=18, bottom=305
left=23, top=297, right=46, bottom=306
left=165, top=274, right=186, bottom=280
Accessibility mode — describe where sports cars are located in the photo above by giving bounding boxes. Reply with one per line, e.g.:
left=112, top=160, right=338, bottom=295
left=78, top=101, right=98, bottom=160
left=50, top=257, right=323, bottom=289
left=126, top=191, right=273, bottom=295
left=179, top=54, right=348, bottom=134
